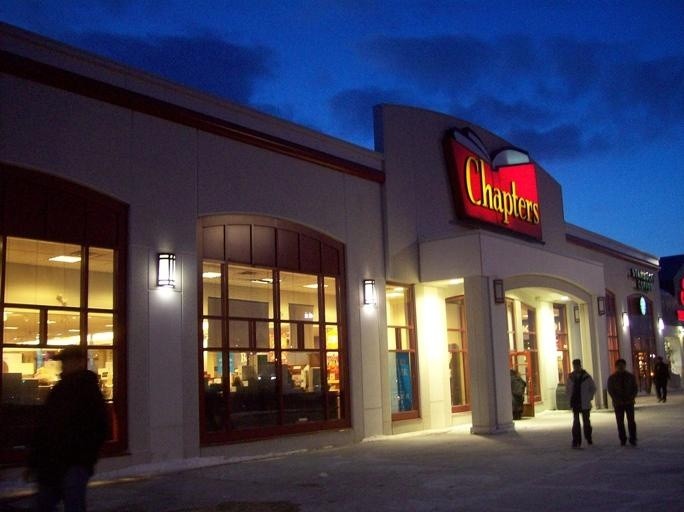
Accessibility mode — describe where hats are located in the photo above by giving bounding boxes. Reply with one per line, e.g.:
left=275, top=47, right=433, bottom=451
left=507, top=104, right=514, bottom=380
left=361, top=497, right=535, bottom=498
left=50, top=345, right=87, bottom=361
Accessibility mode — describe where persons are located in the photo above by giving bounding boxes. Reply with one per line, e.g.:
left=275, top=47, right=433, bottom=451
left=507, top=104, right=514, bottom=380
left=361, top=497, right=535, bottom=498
left=607, top=359, right=639, bottom=447
left=510, top=369, right=526, bottom=420
left=565, top=359, right=596, bottom=447
left=23, top=345, right=112, bottom=512
left=653, top=356, right=671, bottom=403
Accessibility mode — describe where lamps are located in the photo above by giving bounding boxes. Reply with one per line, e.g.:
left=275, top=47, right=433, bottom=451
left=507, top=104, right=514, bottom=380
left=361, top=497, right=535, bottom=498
left=155, top=252, right=175, bottom=288
